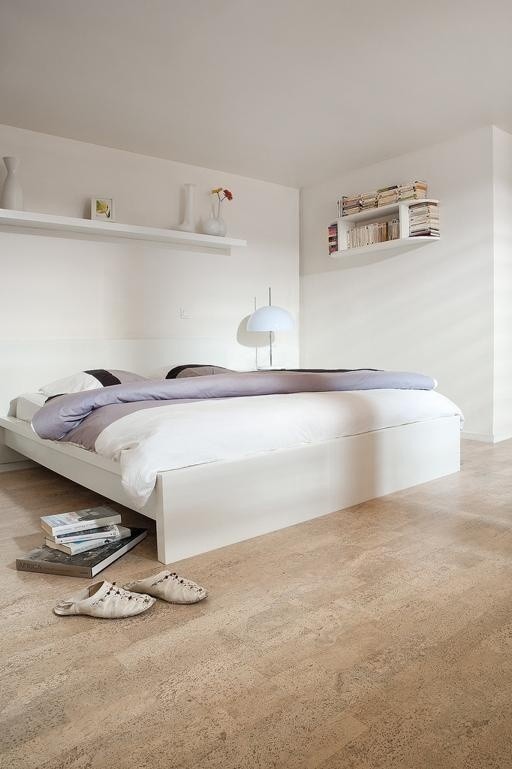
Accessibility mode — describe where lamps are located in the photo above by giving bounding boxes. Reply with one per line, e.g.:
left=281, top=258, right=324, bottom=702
left=247, top=283, right=298, bottom=372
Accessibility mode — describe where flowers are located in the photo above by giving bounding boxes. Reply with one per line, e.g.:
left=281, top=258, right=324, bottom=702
left=204, top=186, right=238, bottom=216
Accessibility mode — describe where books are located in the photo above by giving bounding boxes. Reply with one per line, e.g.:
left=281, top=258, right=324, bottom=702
left=56, top=523, right=119, bottom=544
left=46, top=525, right=131, bottom=555
left=327, top=180, right=442, bottom=255
left=40, top=503, right=122, bottom=536
left=16, top=526, right=147, bottom=577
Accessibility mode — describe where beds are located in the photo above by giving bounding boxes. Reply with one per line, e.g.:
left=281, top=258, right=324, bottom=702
left=3, top=361, right=463, bottom=570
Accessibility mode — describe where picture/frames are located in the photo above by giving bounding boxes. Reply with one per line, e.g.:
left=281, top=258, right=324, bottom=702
left=90, top=194, right=117, bottom=223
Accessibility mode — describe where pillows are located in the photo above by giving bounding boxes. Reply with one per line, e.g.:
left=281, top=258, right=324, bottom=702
left=165, top=366, right=241, bottom=375
left=16, top=368, right=155, bottom=421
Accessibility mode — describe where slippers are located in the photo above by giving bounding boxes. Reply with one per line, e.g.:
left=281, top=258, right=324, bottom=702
left=121, top=570, right=208, bottom=605
left=54, top=580, right=156, bottom=619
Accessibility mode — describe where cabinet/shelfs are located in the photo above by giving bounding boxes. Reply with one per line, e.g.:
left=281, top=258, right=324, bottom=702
left=324, top=198, right=442, bottom=267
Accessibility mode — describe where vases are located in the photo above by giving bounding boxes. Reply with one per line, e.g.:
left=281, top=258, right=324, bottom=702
left=197, top=212, right=227, bottom=239
